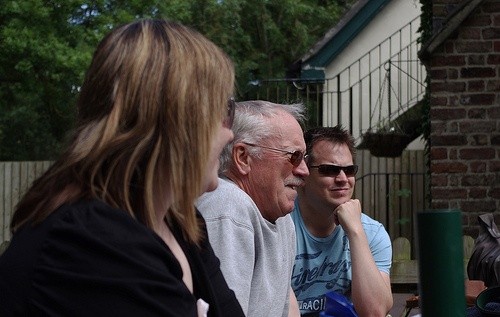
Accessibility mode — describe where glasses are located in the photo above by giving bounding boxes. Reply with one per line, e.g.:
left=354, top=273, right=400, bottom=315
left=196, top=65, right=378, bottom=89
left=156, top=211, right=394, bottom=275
left=243, top=142, right=309, bottom=168
left=223, top=96, right=235, bottom=129
left=308, top=164, right=359, bottom=177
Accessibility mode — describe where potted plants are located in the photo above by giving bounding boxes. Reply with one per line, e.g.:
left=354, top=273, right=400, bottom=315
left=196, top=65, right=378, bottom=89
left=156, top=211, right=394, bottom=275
left=364, top=115, right=409, bottom=157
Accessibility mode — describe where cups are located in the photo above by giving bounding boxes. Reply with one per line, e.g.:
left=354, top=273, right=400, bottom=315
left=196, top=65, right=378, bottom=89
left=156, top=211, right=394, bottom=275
left=476, top=286, right=500, bottom=316
left=412, top=210, right=467, bottom=316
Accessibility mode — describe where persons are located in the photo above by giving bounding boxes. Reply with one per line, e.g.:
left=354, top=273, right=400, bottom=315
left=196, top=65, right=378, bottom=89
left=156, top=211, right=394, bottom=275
left=289, top=123, right=393, bottom=316
left=1, top=18, right=245, bottom=317
left=192, top=101, right=310, bottom=316
